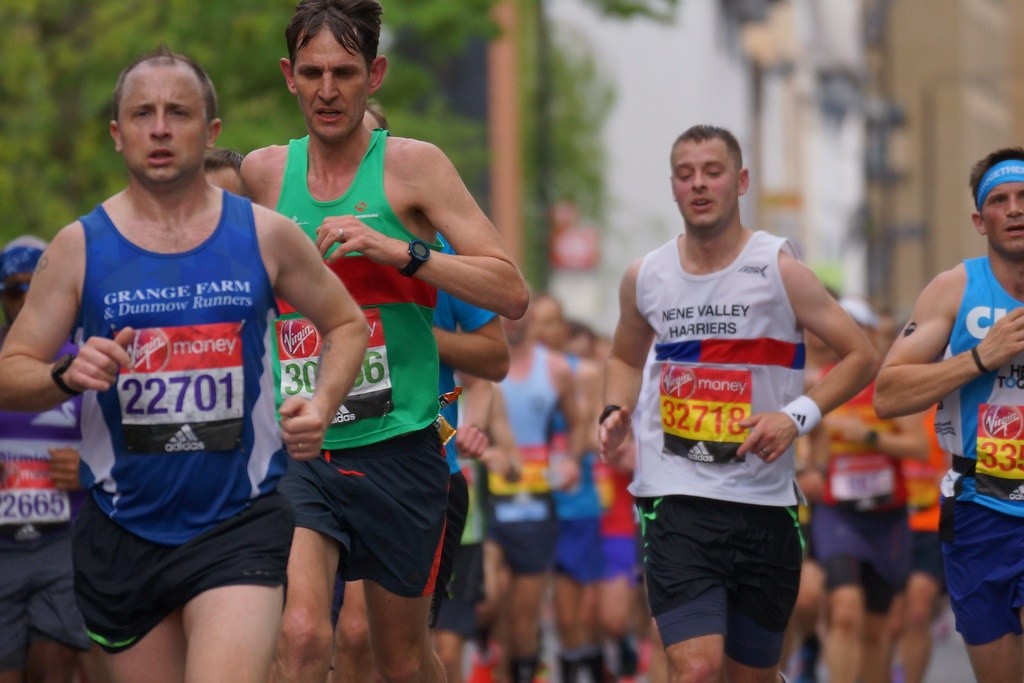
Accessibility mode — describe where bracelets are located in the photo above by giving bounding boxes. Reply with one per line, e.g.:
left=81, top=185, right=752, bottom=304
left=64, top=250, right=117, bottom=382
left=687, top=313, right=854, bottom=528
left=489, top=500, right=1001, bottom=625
left=779, top=395, right=822, bottom=437
left=971, top=346, right=990, bottom=375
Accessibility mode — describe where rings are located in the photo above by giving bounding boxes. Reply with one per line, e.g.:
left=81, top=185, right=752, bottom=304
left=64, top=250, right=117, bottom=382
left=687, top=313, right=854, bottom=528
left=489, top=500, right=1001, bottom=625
left=339, top=229, right=345, bottom=240
left=298, top=443, right=304, bottom=452
left=762, top=448, right=770, bottom=455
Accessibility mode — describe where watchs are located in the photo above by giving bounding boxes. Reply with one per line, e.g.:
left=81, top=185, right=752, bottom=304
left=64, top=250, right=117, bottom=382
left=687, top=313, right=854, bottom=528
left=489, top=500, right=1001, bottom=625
left=398, top=239, right=431, bottom=278
left=598, top=405, right=621, bottom=425
left=51, top=353, right=83, bottom=397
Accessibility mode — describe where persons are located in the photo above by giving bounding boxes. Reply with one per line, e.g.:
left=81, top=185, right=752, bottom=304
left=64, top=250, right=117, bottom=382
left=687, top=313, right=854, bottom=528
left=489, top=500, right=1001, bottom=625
left=872, top=147, right=1024, bottom=683
left=240, top=0, right=532, bottom=683
left=0, top=148, right=951, bottom=683
left=0, top=52, right=372, bottom=683
left=597, top=124, right=881, bottom=683
